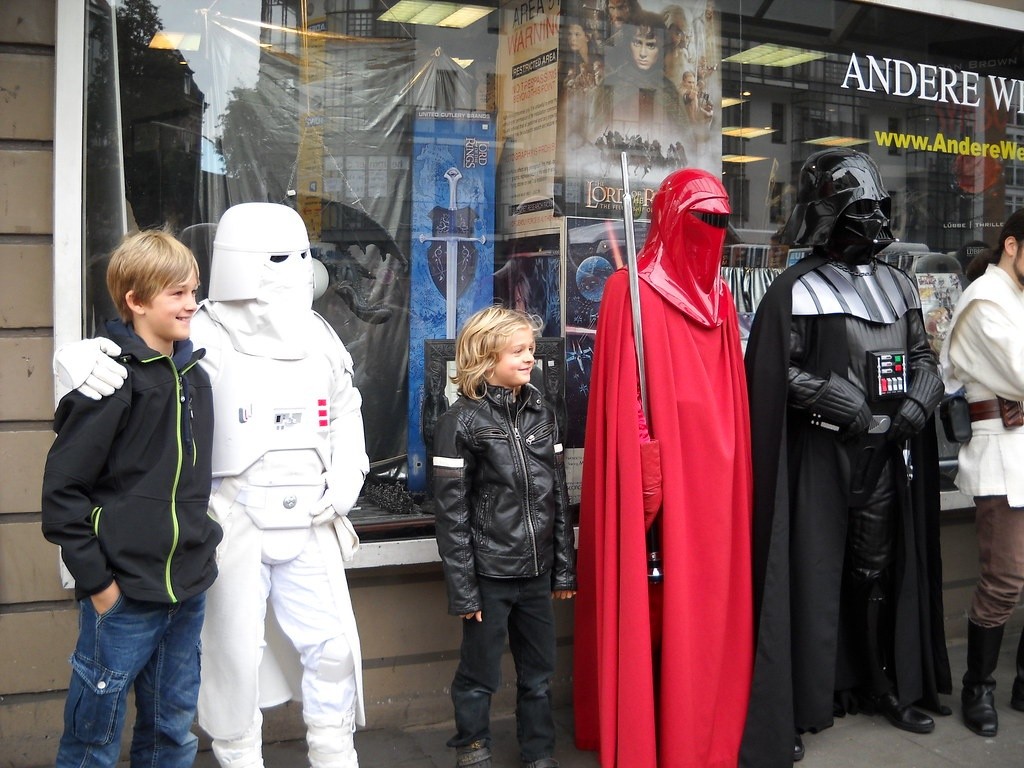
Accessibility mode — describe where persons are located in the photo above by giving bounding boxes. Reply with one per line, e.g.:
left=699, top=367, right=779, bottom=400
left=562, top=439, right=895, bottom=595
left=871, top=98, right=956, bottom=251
left=744, top=147, right=945, bottom=761
left=563, top=1, right=718, bottom=163
left=431, top=305, right=577, bottom=768
left=42, top=230, right=223, bottom=768
left=52, top=202, right=370, bottom=767
left=939, top=207, right=1024, bottom=738
left=574, top=168, right=754, bottom=768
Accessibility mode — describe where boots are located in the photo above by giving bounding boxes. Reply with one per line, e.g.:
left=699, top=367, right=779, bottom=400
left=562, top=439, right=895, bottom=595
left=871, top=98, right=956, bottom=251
left=791, top=727, right=805, bottom=760
left=961, top=615, right=1006, bottom=737
left=846, top=571, right=935, bottom=732
left=1010, top=627, right=1024, bottom=711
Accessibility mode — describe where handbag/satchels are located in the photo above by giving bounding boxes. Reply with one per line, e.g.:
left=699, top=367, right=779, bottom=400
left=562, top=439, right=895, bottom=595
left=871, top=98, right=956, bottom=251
left=939, top=395, right=972, bottom=444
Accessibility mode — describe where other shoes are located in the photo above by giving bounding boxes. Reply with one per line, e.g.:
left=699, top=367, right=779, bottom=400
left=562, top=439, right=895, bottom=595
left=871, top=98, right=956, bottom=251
left=521, top=758, right=560, bottom=768
left=456, top=739, right=492, bottom=768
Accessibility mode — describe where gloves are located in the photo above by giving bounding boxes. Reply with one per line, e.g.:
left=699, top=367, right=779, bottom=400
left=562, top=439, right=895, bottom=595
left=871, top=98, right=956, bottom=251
left=310, top=491, right=339, bottom=527
left=885, top=368, right=945, bottom=443
left=801, top=370, right=872, bottom=441
left=640, top=438, right=663, bottom=534
left=70, top=336, right=128, bottom=400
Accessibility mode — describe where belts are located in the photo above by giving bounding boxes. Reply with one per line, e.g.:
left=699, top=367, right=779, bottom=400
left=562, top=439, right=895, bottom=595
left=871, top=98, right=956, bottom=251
left=968, top=399, right=1001, bottom=422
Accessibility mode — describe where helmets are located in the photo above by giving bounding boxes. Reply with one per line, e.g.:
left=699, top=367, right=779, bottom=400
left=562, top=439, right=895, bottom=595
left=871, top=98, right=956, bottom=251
left=616, top=168, right=732, bottom=327
left=770, top=146, right=899, bottom=255
left=202, top=203, right=315, bottom=361
